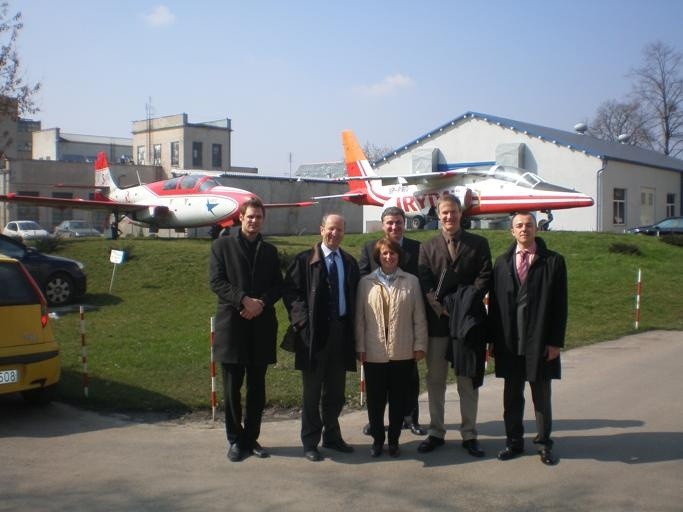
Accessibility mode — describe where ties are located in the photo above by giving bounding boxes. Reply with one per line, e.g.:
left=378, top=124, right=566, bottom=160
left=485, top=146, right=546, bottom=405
left=447, top=238, right=457, bottom=261
left=323, top=252, right=340, bottom=318
left=517, top=250, right=530, bottom=283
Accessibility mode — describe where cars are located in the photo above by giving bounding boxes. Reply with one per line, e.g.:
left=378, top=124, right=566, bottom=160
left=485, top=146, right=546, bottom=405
left=55, top=220, right=100, bottom=240
left=0, top=254, right=62, bottom=409
left=0, top=234, right=87, bottom=306
left=2, top=220, right=49, bottom=240
left=623, top=216, right=683, bottom=237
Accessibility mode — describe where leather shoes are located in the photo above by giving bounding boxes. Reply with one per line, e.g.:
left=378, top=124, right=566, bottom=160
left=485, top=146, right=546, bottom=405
left=497, top=444, right=524, bottom=461
left=417, top=435, right=445, bottom=453
left=322, top=439, right=354, bottom=453
left=304, top=447, right=322, bottom=462
left=404, top=422, right=428, bottom=435
left=369, top=443, right=383, bottom=457
left=462, top=439, right=486, bottom=458
left=539, top=447, right=557, bottom=466
left=388, top=447, right=401, bottom=458
left=226, top=439, right=251, bottom=462
left=362, top=422, right=372, bottom=436
left=250, top=439, right=270, bottom=458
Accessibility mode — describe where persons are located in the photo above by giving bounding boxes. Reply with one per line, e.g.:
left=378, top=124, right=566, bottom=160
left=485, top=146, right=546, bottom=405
left=488, top=210, right=568, bottom=466
left=278, top=213, right=360, bottom=462
left=417, top=194, right=493, bottom=458
left=355, top=239, right=428, bottom=459
left=208, top=199, right=284, bottom=462
left=359, top=206, right=427, bottom=437
